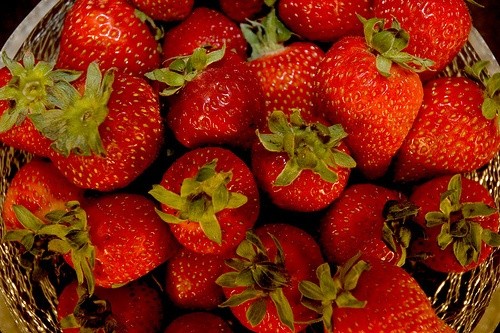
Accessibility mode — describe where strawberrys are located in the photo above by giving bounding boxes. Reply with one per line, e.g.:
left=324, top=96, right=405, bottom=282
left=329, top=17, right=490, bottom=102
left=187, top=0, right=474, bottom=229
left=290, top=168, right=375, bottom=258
left=0, top=0, right=500, bottom=333
